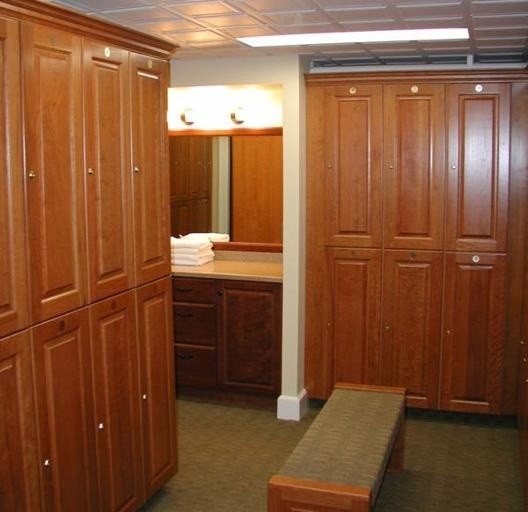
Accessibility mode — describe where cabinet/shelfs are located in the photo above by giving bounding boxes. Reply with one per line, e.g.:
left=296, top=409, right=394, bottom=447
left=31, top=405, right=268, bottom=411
left=172, top=277, right=214, bottom=391
left=0, top=0, right=182, bottom=510
left=167, top=137, right=211, bottom=233
left=304, top=67, right=527, bottom=418
left=215, top=278, right=281, bottom=393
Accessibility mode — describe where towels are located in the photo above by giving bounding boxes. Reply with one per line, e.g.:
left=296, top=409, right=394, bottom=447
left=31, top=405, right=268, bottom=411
left=184, top=231, right=232, bottom=241
left=169, top=235, right=215, bottom=268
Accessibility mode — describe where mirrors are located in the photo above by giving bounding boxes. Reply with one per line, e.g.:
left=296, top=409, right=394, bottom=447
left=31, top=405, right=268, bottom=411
left=167, top=129, right=283, bottom=250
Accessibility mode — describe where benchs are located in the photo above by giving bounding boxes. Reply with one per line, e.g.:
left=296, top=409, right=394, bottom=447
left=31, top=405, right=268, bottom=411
left=267, top=380, right=408, bottom=510
